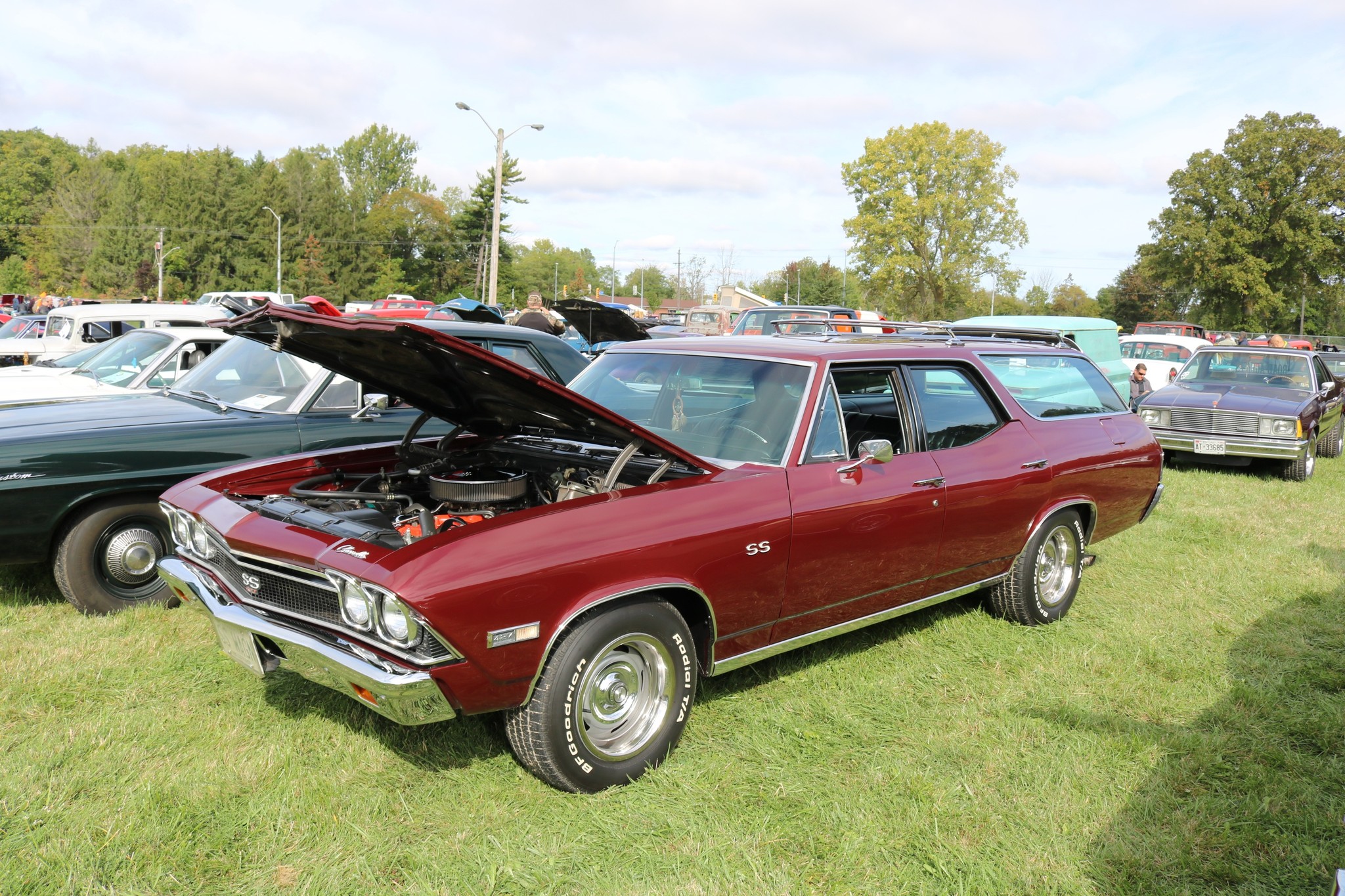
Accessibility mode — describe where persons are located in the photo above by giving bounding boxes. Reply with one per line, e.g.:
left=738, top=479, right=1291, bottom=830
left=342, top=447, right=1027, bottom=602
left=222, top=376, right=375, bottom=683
left=1204, top=330, right=1322, bottom=372
left=13, top=292, right=77, bottom=315
left=140, top=296, right=199, bottom=305
left=505, top=291, right=566, bottom=368
left=1129, top=364, right=1152, bottom=401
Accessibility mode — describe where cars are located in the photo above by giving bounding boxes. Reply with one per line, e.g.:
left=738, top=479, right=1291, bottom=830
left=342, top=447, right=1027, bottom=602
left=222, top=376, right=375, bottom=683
left=1135, top=343, right=1345, bottom=483
left=150, top=299, right=1164, bottom=798
left=1, top=318, right=749, bottom=618
left=0, top=289, right=1345, bottom=442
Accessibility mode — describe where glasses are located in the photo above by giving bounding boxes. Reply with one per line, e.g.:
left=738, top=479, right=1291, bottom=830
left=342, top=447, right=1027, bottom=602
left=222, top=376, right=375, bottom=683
left=1137, top=371, right=1146, bottom=376
left=46, top=301, right=49, bottom=303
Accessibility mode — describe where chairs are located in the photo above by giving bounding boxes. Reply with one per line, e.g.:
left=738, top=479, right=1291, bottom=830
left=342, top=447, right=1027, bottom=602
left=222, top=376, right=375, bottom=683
left=821, top=431, right=903, bottom=459
left=180, top=349, right=206, bottom=370
left=1281, top=375, right=1309, bottom=384
left=1210, top=372, right=1237, bottom=381
left=691, top=417, right=775, bottom=444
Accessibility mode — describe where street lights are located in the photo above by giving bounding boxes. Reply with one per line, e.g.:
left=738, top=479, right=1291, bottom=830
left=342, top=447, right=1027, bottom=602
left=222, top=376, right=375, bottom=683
left=454, top=101, right=546, bottom=304
left=777, top=273, right=789, bottom=305
left=554, top=262, right=559, bottom=307
left=841, top=247, right=848, bottom=307
left=156, top=245, right=181, bottom=300
left=797, top=269, right=802, bottom=306
left=260, top=205, right=286, bottom=293
left=611, top=239, right=620, bottom=304
left=482, top=252, right=502, bottom=303
left=626, top=258, right=644, bottom=309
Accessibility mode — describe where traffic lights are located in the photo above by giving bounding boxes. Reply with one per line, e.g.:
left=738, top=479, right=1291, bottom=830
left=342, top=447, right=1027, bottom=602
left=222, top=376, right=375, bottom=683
left=783, top=293, right=787, bottom=302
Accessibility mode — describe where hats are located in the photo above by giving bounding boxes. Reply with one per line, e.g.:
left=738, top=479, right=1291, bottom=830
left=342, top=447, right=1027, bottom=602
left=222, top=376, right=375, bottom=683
left=528, top=291, right=542, bottom=302
left=1222, top=332, right=1225, bottom=335
left=60, top=298, right=64, bottom=301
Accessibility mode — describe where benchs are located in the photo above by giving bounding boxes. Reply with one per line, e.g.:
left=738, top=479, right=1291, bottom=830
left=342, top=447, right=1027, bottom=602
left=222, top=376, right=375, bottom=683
left=812, top=411, right=989, bottom=457
